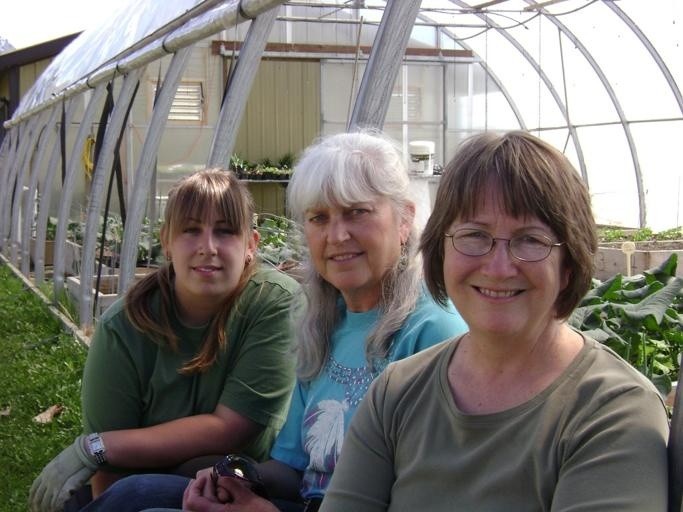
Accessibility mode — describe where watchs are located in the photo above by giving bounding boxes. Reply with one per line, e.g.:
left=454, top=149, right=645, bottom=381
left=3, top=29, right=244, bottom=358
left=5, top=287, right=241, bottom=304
left=86, top=432, right=106, bottom=466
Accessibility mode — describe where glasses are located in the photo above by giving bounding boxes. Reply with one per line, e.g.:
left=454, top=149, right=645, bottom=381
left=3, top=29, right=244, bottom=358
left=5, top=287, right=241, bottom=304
left=443, top=229, right=565, bottom=262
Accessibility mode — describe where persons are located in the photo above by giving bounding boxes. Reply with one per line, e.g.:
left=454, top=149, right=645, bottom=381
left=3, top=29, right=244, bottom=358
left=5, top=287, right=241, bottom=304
left=27, top=168, right=308, bottom=511
left=318, top=130, right=669, bottom=512
left=140, top=132, right=470, bottom=511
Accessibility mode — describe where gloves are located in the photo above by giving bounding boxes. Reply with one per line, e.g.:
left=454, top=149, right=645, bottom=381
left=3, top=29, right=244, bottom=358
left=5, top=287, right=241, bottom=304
left=28, top=435, right=97, bottom=512
